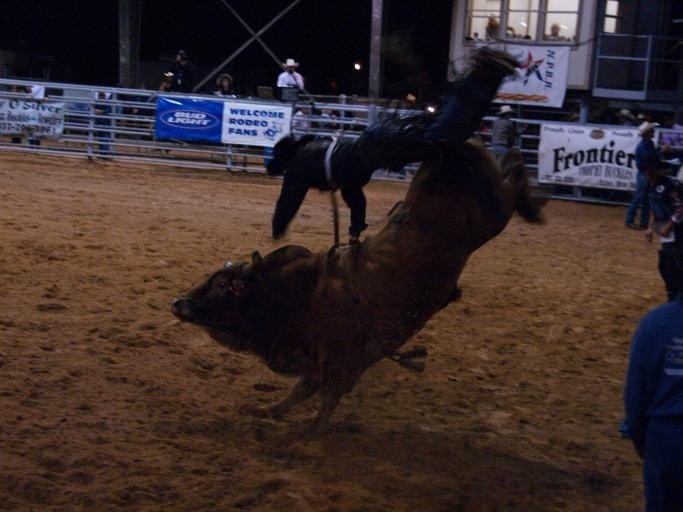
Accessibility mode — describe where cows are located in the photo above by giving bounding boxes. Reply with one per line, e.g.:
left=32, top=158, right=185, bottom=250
left=170, top=137, right=554, bottom=440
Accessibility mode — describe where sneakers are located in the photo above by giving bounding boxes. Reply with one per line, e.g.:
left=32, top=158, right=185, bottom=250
left=623, top=222, right=650, bottom=231
left=473, top=53, right=518, bottom=76
left=478, top=45, right=521, bottom=69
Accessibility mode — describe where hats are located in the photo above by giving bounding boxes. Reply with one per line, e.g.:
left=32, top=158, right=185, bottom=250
left=214, top=74, right=232, bottom=87
left=281, top=57, right=298, bottom=68
left=637, top=121, right=660, bottom=135
left=496, top=104, right=515, bottom=116
left=265, top=137, right=308, bottom=178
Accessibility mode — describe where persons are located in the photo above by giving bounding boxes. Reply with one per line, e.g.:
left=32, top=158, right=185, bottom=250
left=26, top=84, right=52, bottom=148
left=618, top=240, right=683, bottom=512
left=624, top=120, right=670, bottom=231
left=85, top=92, right=115, bottom=160
left=493, top=105, right=531, bottom=183
left=544, top=24, right=570, bottom=42
left=483, top=17, right=513, bottom=40
left=4, top=84, right=28, bottom=144
left=638, top=149, right=683, bottom=248
